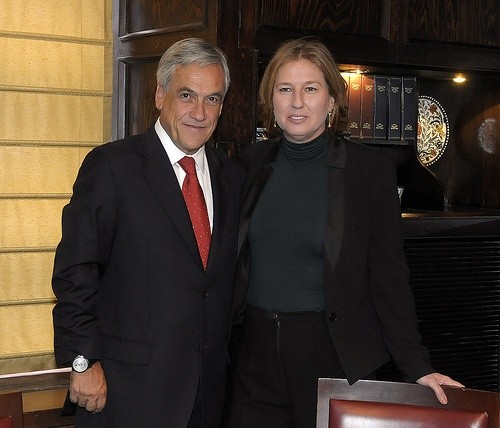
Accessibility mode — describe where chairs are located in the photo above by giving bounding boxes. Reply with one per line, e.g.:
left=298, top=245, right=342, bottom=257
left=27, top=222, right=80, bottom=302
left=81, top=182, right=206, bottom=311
left=317, top=377, right=500, bottom=428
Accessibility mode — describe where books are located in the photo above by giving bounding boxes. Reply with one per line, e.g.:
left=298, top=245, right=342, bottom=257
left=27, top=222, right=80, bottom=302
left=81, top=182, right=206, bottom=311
left=337, top=75, right=418, bottom=139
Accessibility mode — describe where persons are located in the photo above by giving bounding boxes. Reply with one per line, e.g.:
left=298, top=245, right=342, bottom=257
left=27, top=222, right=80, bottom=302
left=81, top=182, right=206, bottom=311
left=51, top=38, right=245, bottom=428
left=227, top=34, right=467, bottom=428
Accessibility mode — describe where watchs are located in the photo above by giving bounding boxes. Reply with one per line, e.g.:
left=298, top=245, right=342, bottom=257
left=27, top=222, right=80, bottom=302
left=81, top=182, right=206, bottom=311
left=72, top=355, right=98, bottom=373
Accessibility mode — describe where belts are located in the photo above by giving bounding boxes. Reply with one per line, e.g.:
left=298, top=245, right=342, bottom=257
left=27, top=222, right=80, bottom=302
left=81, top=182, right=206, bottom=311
left=248, top=304, right=333, bottom=327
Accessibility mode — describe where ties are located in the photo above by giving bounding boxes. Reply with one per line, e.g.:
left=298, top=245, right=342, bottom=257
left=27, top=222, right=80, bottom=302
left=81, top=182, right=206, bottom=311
left=177, top=156, right=211, bottom=272
